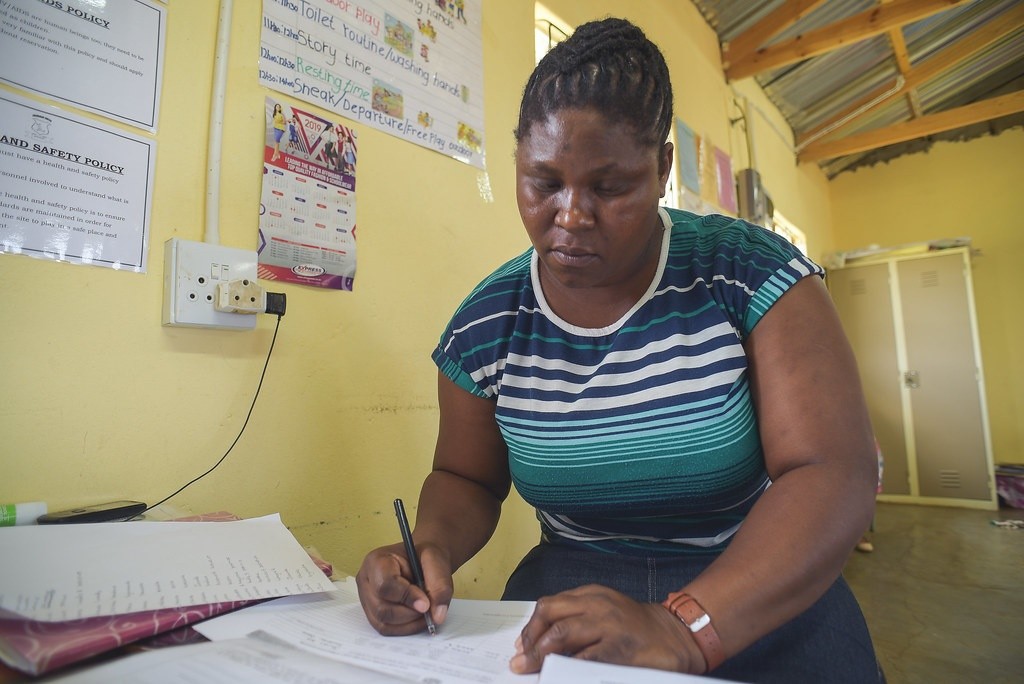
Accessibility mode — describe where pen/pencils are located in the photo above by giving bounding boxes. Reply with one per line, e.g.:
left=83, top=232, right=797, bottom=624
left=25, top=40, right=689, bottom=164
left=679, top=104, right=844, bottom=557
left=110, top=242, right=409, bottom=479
left=393, top=497, right=436, bottom=636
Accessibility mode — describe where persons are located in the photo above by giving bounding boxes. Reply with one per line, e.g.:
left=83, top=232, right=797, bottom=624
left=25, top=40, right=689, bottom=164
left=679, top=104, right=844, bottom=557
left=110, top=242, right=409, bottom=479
left=335, top=132, right=346, bottom=171
left=270, top=103, right=286, bottom=162
left=355, top=17, right=879, bottom=684
left=344, top=136, right=357, bottom=177
left=285, top=114, right=300, bottom=154
left=320, top=126, right=337, bottom=169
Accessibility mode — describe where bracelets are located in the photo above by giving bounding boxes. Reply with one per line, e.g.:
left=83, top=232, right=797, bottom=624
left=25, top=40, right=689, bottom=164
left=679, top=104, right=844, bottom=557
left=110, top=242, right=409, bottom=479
left=662, top=591, right=727, bottom=673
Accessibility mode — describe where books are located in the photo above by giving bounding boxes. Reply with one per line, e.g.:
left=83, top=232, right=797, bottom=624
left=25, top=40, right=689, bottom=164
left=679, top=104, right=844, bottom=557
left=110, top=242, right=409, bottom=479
left=0, top=510, right=332, bottom=676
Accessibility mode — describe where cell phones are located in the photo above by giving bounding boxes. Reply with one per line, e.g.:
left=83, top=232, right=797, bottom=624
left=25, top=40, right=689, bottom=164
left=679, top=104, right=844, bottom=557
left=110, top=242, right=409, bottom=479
left=37, top=501, right=147, bottom=525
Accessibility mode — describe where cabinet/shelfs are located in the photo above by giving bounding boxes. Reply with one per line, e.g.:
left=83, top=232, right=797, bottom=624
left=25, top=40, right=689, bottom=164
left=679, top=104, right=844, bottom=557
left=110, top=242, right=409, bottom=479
left=823, top=245, right=997, bottom=511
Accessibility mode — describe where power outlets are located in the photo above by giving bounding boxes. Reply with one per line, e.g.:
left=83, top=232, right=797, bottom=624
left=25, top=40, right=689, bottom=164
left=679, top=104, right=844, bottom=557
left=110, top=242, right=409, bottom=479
left=214, top=277, right=267, bottom=314
left=160, top=236, right=258, bottom=332
